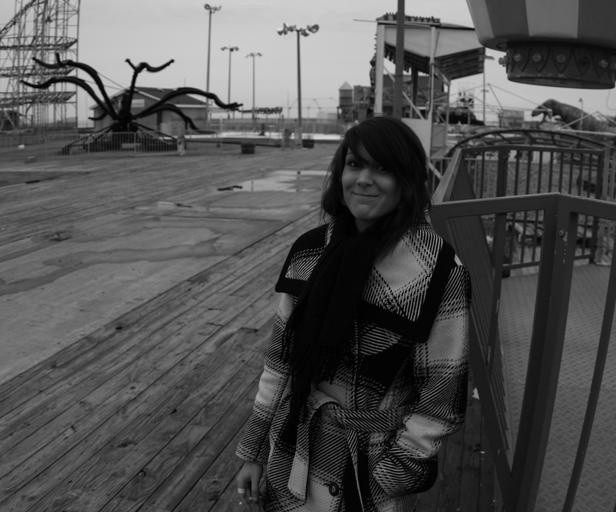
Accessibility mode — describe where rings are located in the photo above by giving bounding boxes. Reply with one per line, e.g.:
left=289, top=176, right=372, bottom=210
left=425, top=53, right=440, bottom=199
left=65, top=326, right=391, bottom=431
left=236, top=488, right=245, bottom=494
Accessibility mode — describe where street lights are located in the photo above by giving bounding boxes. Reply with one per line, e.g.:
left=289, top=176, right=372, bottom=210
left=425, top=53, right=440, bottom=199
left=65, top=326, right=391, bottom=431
left=243, top=51, right=263, bottom=118
left=480, top=51, right=496, bottom=121
left=218, top=44, right=239, bottom=119
left=277, top=21, right=320, bottom=134
left=201, top=2, right=222, bottom=120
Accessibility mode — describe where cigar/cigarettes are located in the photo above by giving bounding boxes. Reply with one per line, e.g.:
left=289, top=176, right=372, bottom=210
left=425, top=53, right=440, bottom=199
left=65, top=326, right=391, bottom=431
left=238, top=496, right=261, bottom=505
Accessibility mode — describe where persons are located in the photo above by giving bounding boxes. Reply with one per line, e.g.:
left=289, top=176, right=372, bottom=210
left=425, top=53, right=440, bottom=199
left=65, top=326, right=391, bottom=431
left=235, top=116, right=472, bottom=511
